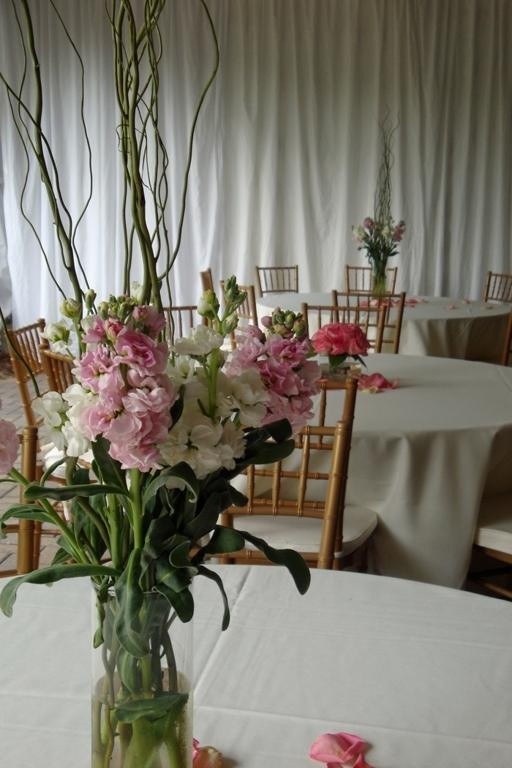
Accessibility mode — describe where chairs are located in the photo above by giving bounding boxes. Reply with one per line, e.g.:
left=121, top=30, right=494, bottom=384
left=39, top=343, right=82, bottom=393
left=464, top=491, right=511, bottom=602
left=218, top=369, right=379, bottom=569
left=4, top=318, right=52, bottom=575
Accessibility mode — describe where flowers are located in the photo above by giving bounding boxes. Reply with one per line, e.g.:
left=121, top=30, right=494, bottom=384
left=348, top=100, right=407, bottom=296
left=0, top=1, right=370, bottom=767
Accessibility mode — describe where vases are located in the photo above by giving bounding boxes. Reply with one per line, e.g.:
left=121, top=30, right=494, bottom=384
left=89, top=587, right=194, bottom=766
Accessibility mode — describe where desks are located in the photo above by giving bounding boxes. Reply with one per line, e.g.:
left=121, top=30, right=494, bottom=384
left=217, top=352, right=511, bottom=589
left=247, top=291, right=512, bottom=365
left=1, top=565, right=511, bottom=768
